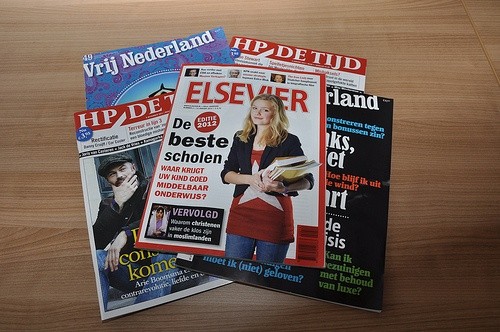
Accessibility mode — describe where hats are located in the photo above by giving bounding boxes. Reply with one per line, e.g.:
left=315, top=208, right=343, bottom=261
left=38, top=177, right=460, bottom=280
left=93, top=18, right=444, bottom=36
left=97, top=152, right=132, bottom=174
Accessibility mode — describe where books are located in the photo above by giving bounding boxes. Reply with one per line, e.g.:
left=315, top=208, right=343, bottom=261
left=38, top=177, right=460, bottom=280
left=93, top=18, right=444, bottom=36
left=226, top=36, right=368, bottom=93
left=134, top=62, right=332, bottom=268
left=73, top=90, right=236, bottom=321
left=173, top=85, right=395, bottom=312
left=83, top=25, right=234, bottom=112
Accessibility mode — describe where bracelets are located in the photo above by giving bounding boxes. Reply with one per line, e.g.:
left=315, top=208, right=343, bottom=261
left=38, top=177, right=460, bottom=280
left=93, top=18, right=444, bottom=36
left=284, top=187, right=289, bottom=193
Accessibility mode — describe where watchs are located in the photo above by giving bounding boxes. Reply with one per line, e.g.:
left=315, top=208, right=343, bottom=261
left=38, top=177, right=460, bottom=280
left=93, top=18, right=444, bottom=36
left=111, top=200, right=121, bottom=212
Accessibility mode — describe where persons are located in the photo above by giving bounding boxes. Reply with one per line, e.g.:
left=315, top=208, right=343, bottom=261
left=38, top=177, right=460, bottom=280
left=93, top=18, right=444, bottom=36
left=220, top=94, right=315, bottom=264
left=147, top=207, right=169, bottom=241
left=228, top=69, right=240, bottom=79
left=273, top=74, right=283, bottom=84
left=186, top=69, right=198, bottom=78
left=91, top=154, right=177, bottom=312
